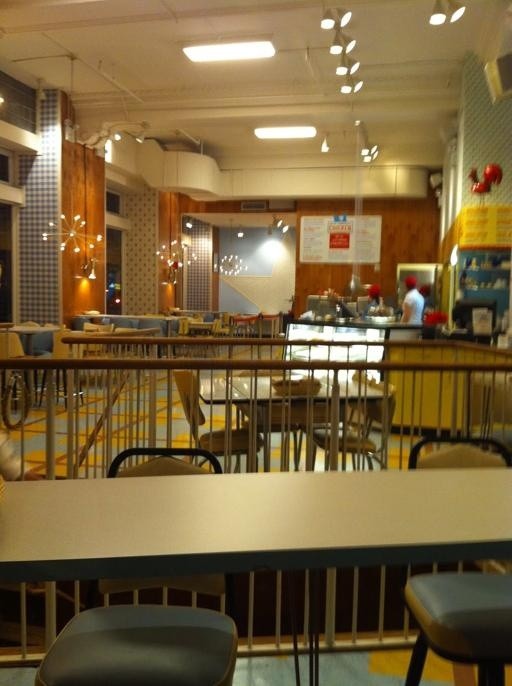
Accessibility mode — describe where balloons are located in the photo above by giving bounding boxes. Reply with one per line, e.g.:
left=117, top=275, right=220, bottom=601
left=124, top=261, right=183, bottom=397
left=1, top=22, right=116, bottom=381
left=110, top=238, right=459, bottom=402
left=471, top=182, right=490, bottom=193
left=483, top=163, right=501, bottom=186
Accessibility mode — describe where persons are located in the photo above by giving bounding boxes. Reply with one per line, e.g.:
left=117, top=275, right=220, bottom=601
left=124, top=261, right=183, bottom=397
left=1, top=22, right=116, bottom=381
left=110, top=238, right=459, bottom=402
left=400, top=278, right=425, bottom=325
left=360, top=283, right=382, bottom=317
left=419, top=284, right=434, bottom=308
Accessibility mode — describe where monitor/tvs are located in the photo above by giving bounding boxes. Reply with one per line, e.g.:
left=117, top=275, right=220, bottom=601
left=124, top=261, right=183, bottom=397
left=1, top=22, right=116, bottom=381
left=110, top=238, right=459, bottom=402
left=335, top=298, right=359, bottom=319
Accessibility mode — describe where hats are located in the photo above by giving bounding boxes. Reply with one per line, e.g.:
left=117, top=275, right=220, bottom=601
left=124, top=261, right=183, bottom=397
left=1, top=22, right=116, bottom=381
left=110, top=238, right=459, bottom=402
left=369, top=285, right=380, bottom=297
left=405, top=277, right=417, bottom=287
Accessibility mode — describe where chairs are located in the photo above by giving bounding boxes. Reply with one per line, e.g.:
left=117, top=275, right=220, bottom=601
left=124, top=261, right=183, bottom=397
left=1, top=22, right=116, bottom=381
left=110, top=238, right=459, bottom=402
left=0, top=309, right=282, bottom=409
left=407, top=435, right=512, bottom=468
left=105, top=446, right=225, bottom=476
left=173, top=369, right=396, bottom=472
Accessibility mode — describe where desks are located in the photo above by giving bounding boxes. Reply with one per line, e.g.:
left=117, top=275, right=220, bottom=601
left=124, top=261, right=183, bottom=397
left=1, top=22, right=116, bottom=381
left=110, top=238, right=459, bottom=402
left=0, top=465, right=512, bottom=582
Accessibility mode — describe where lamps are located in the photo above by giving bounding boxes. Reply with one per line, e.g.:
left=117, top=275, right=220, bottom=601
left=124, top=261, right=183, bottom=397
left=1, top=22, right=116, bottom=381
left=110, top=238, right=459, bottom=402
left=113, top=130, right=147, bottom=143
left=429, top=0, right=466, bottom=26
left=156, top=240, right=198, bottom=286
left=42, top=214, right=103, bottom=280
left=216, top=254, right=247, bottom=276
left=320, top=6, right=379, bottom=163
left=184, top=215, right=289, bottom=238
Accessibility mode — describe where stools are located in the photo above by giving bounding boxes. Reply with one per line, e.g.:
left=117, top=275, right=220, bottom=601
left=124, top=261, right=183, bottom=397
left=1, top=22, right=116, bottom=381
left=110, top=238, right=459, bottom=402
left=399, top=571, right=510, bottom=685
left=35, top=603, right=243, bottom=685
